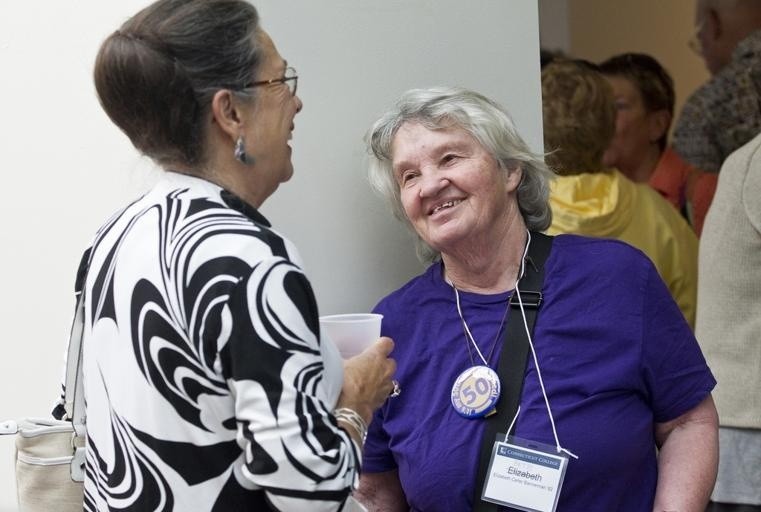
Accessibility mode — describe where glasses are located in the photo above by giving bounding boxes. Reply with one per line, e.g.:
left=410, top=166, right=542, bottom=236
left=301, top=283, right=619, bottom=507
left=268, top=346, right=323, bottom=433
left=210, top=63, right=301, bottom=129
left=685, top=17, right=709, bottom=60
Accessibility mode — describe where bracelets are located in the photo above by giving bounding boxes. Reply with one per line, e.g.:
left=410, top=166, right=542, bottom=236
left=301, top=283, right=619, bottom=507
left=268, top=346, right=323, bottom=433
left=333, top=408, right=369, bottom=445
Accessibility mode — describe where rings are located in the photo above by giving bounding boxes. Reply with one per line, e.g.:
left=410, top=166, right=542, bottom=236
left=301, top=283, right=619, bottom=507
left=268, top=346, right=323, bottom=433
left=391, top=379, right=402, bottom=399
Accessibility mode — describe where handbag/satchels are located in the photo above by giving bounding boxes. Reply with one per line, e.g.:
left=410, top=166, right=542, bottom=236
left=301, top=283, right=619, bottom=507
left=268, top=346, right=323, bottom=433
left=0, top=190, right=148, bottom=512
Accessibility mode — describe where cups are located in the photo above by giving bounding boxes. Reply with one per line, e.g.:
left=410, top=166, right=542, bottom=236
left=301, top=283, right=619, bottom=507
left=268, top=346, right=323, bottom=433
left=317, top=314, right=383, bottom=413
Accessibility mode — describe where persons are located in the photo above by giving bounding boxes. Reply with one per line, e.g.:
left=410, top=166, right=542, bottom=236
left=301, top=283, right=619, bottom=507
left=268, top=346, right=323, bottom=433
left=671, top=1, right=761, bottom=172
left=543, top=57, right=702, bottom=334
left=343, top=81, right=722, bottom=512
left=599, top=50, right=720, bottom=238
left=59, top=0, right=399, bottom=511
left=693, top=133, right=760, bottom=512
